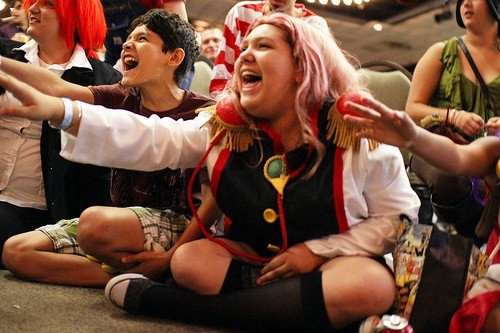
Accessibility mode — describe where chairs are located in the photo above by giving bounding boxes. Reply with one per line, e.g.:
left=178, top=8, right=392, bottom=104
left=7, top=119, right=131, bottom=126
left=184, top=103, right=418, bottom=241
left=355, top=59, right=414, bottom=111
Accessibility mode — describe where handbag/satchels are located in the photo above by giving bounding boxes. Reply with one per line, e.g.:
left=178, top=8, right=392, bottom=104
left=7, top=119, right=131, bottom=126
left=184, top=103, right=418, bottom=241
left=392, top=213, right=474, bottom=333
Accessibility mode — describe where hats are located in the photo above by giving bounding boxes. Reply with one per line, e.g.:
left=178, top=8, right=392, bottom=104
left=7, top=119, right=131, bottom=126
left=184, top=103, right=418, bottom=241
left=456, top=0, right=500, bottom=28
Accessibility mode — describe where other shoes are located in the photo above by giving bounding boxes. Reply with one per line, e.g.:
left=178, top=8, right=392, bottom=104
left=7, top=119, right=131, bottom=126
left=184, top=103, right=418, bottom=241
left=103, top=272, right=178, bottom=319
